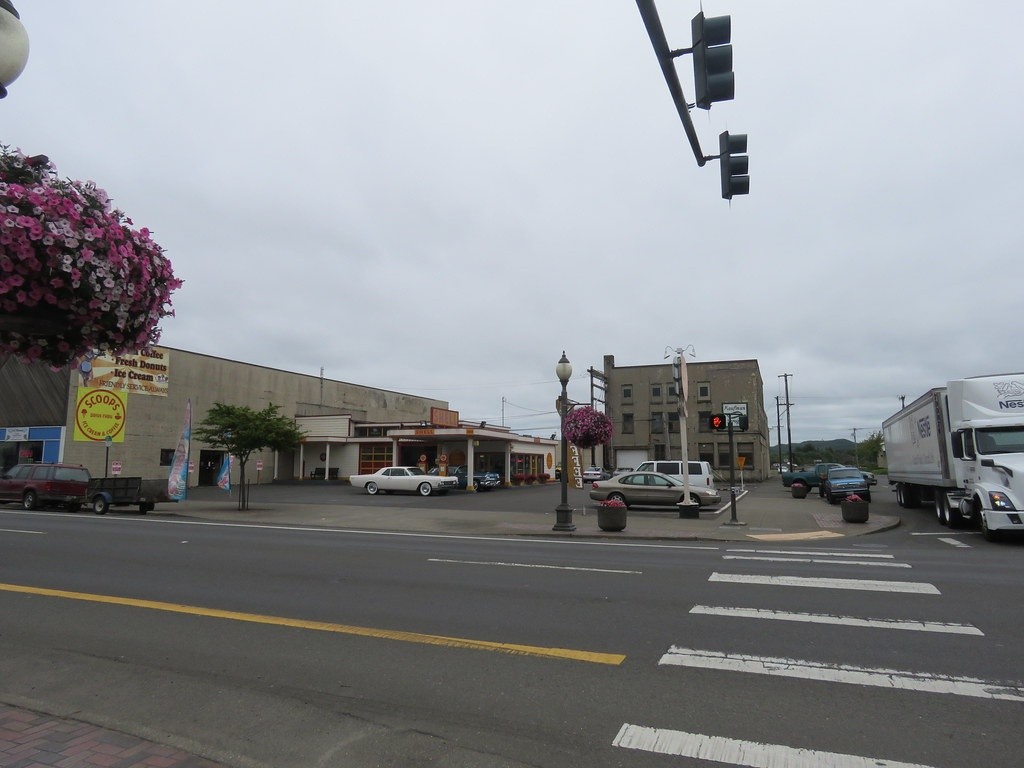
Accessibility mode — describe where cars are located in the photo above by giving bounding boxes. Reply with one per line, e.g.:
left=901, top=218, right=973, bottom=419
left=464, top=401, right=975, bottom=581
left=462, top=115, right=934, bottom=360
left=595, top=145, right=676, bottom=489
left=772, top=463, right=798, bottom=473
left=428, top=466, right=458, bottom=475
left=348, top=466, right=459, bottom=496
left=590, top=471, right=721, bottom=509
left=612, top=467, right=633, bottom=478
left=582, top=466, right=611, bottom=484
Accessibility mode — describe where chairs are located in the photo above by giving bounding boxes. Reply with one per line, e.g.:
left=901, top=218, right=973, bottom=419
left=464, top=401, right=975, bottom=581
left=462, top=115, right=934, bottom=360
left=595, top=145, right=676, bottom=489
left=648, top=478, right=656, bottom=486
left=980, top=435, right=997, bottom=452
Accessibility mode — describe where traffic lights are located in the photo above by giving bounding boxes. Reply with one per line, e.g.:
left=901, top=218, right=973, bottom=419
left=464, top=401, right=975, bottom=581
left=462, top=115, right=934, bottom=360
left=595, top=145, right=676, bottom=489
left=691, top=11, right=736, bottom=109
left=708, top=415, right=726, bottom=430
left=719, top=131, right=749, bottom=200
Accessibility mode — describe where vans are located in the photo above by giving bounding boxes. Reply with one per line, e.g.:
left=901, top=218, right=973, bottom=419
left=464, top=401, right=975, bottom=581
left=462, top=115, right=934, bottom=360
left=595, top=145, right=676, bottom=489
left=0, top=462, right=92, bottom=513
left=620, top=460, right=714, bottom=489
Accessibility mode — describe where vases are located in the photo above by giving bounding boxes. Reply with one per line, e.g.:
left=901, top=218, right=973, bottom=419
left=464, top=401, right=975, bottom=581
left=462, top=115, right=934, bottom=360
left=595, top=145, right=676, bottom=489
left=791, top=486, right=808, bottom=499
left=528, top=481, right=533, bottom=485
left=539, top=479, right=547, bottom=484
left=514, top=481, right=521, bottom=486
left=596, top=506, right=628, bottom=532
left=840, top=500, right=869, bottom=523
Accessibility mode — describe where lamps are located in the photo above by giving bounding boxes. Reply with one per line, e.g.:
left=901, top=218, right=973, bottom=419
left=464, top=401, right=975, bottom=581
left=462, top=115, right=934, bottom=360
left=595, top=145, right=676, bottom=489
left=420, top=420, right=427, bottom=429
left=479, top=421, right=486, bottom=429
left=550, top=434, right=556, bottom=440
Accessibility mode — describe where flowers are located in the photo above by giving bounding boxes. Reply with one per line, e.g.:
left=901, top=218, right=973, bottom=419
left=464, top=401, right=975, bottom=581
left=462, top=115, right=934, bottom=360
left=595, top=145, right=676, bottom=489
left=525, top=474, right=537, bottom=481
left=562, top=405, right=617, bottom=450
left=600, top=500, right=626, bottom=507
left=790, top=483, right=804, bottom=487
left=537, top=473, right=550, bottom=480
left=847, top=494, right=864, bottom=502
left=512, top=473, right=525, bottom=482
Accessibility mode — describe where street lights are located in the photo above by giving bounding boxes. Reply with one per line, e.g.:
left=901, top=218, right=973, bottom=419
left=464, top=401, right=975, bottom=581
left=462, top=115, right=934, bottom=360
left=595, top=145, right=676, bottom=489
left=552, top=350, right=578, bottom=532
left=663, top=344, right=699, bottom=507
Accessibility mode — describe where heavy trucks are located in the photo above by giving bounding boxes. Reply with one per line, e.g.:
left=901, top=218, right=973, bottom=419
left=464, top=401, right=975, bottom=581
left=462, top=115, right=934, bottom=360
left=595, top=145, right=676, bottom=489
left=881, top=373, right=1024, bottom=541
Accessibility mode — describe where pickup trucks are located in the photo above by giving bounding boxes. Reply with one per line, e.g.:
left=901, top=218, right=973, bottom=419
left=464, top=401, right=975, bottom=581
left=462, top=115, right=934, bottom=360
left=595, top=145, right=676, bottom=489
left=818, top=467, right=871, bottom=504
left=782, top=463, right=878, bottom=494
left=449, top=465, right=501, bottom=492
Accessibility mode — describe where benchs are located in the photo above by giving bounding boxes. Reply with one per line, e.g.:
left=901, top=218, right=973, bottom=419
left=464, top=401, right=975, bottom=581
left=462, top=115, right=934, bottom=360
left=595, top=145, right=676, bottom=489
left=310, top=468, right=339, bottom=480
